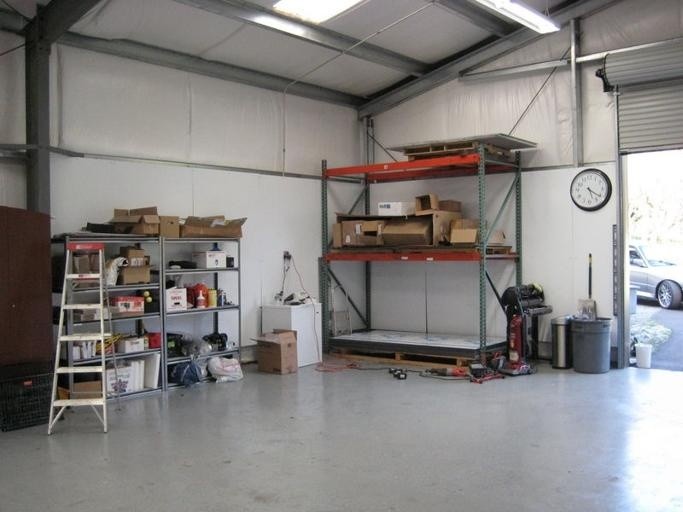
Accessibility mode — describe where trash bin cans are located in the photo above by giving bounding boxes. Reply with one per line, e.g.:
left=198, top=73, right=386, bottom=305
left=549, top=315, right=571, bottom=369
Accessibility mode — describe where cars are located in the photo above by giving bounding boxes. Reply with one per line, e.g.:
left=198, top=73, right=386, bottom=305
left=628, top=241, right=683, bottom=310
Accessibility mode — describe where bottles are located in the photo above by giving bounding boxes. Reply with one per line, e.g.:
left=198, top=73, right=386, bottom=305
left=143, top=331, right=149, bottom=351
left=196, top=289, right=205, bottom=310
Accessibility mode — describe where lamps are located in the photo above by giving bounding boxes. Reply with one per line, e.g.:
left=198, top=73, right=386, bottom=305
left=467, top=0, right=560, bottom=37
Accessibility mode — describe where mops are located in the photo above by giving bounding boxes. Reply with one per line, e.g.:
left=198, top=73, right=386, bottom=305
left=575, top=253, right=596, bottom=319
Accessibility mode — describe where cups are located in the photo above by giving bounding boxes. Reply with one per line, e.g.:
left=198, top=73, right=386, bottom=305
left=207, top=289, right=217, bottom=308
left=218, top=292, right=226, bottom=307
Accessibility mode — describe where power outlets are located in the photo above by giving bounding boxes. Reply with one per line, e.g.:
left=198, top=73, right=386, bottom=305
left=282, top=249, right=291, bottom=265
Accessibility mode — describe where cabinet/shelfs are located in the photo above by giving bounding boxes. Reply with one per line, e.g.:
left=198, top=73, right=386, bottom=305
left=133, top=236, right=242, bottom=393
left=258, top=298, right=322, bottom=368
left=50, top=233, right=164, bottom=414
left=316, top=143, right=523, bottom=371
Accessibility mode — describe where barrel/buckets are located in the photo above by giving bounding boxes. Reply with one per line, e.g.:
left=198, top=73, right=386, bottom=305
left=635, top=343, right=652, bottom=368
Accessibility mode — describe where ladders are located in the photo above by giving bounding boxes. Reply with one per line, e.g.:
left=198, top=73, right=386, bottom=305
left=47, top=241, right=108, bottom=436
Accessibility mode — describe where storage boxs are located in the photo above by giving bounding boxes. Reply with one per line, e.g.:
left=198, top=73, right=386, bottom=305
left=52, top=244, right=188, bottom=323
left=94, top=363, right=134, bottom=400
left=190, top=250, right=227, bottom=269
left=329, top=190, right=507, bottom=248
left=115, top=335, right=144, bottom=355
left=104, top=206, right=247, bottom=240
left=248, top=327, right=298, bottom=376
left=68, top=378, right=102, bottom=400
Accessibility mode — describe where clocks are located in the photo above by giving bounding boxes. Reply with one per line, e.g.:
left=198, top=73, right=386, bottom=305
left=569, top=167, right=613, bottom=213
